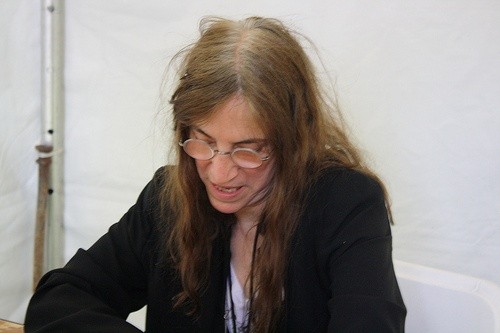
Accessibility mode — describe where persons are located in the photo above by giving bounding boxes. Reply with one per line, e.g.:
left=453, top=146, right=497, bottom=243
left=21, top=12, right=410, bottom=333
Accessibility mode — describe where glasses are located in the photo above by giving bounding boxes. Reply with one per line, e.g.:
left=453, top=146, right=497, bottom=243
left=175, top=136, right=277, bottom=169
